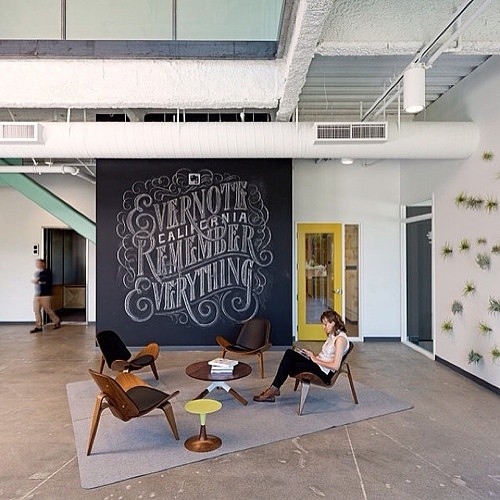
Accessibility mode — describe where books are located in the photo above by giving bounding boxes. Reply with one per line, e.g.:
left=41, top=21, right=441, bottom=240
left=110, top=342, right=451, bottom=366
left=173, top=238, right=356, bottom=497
left=292, top=340, right=311, bottom=360
left=208, top=357, right=239, bottom=374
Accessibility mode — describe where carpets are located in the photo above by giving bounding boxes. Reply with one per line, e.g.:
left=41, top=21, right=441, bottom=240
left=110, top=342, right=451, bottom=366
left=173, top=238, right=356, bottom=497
left=65, top=351, right=414, bottom=489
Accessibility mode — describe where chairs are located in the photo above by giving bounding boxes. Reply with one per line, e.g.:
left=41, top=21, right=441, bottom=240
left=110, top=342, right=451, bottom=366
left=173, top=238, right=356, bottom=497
left=290, top=341, right=359, bottom=415
left=86, top=369, right=180, bottom=456
left=95, top=329, right=160, bottom=381
left=216, top=318, right=272, bottom=380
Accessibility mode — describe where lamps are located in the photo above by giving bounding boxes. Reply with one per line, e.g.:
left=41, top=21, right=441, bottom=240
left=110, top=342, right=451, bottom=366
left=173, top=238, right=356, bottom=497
left=403, top=64, right=426, bottom=114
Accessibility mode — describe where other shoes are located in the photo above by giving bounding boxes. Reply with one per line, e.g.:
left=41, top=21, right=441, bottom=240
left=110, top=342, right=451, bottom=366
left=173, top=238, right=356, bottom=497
left=259, top=385, right=280, bottom=400
left=254, top=392, right=275, bottom=402
left=52, top=320, right=62, bottom=329
left=30, top=326, right=42, bottom=333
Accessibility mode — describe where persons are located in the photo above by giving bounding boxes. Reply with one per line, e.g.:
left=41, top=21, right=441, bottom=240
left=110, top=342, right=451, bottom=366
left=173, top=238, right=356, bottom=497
left=29, top=259, right=61, bottom=333
left=253, top=310, right=350, bottom=402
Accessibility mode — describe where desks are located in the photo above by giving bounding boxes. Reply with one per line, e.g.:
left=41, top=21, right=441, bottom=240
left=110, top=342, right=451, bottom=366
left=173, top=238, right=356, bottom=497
left=185, top=360, right=252, bottom=405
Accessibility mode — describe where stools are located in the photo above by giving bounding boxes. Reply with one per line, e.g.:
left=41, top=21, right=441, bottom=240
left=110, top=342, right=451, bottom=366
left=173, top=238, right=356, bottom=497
left=185, top=398, right=222, bottom=452
